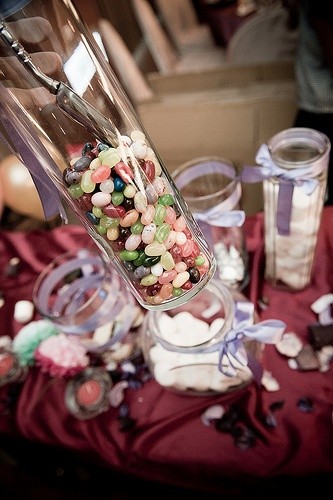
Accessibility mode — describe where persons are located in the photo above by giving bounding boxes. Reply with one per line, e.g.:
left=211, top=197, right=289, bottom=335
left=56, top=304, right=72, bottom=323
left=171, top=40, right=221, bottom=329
left=275, top=0, right=333, bottom=207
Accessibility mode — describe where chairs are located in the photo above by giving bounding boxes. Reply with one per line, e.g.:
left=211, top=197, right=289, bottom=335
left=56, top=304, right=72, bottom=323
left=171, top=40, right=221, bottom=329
left=131, top=0, right=287, bottom=75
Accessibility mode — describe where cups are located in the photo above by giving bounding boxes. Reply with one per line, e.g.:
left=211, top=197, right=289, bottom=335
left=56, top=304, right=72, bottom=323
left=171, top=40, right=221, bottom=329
left=170, top=154, right=252, bottom=292
left=0, top=0, right=217, bottom=311
left=262, top=127, right=331, bottom=291
left=136, top=278, right=262, bottom=396
left=31, top=245, right=137, bottom=356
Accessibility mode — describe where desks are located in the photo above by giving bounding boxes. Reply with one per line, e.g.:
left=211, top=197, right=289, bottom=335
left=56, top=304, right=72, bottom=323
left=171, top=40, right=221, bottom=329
left=0, top=206, right=333, bottom=500
